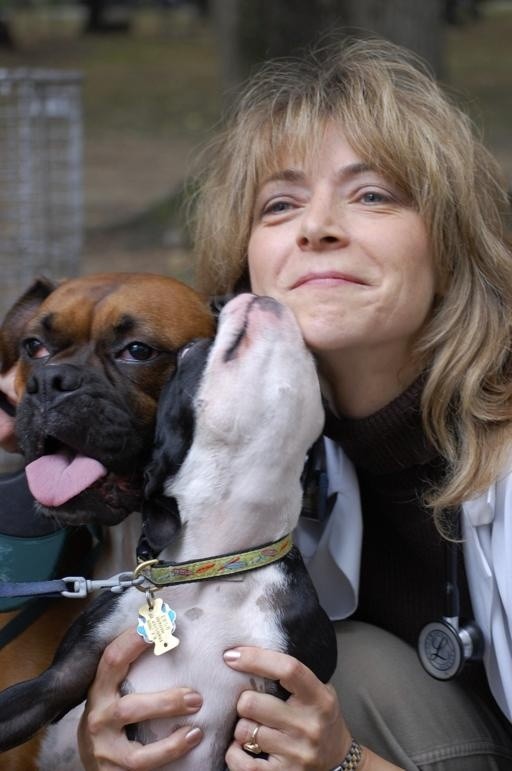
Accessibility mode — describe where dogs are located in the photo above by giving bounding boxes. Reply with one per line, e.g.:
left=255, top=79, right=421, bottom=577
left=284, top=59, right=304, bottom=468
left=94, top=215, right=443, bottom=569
left=2, top=270, right=217, bottom=771
left=2, top=291, right=340, bottom=771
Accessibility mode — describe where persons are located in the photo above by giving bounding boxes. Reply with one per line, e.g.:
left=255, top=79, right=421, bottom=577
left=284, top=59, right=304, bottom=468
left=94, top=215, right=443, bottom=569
left=29, top=30, right=512, bottom=771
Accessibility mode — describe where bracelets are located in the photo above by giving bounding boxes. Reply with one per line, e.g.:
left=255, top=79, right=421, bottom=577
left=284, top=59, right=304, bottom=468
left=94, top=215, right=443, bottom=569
left=330, top=736, right=362, bottom=771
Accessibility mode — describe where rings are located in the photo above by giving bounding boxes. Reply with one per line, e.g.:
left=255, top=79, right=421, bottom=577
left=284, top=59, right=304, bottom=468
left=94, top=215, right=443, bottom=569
left=242, top=724, right=263, bottom=756
